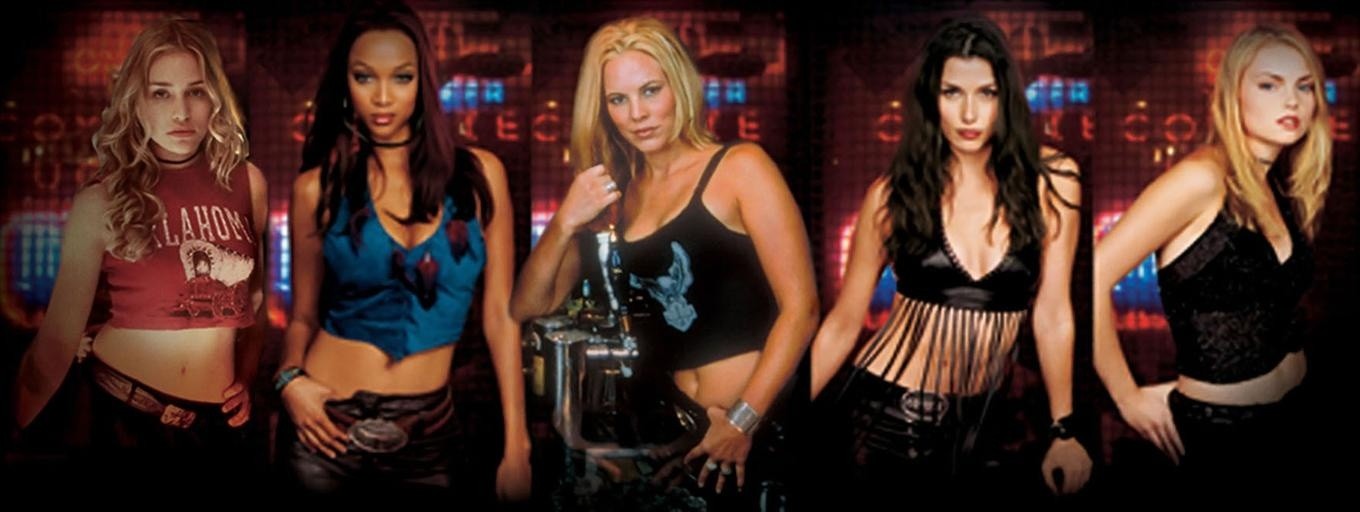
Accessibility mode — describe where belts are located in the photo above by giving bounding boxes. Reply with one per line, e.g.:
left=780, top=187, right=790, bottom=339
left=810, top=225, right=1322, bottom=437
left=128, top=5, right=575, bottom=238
left=842, top=364, right=989, bottom=426
left=324, top=385, right=454, bottom=453
left=94, top=366, right=197, bottom=429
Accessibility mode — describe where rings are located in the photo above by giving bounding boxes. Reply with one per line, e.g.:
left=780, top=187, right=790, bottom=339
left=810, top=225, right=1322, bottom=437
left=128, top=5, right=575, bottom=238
left=606, top=182, right=618, bottom=195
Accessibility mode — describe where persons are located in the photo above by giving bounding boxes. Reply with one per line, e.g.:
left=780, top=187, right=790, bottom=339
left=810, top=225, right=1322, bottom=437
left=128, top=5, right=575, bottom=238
left=811, top=17, right=1091, bottom=508
left=509, top=17, right=820, bottom=511
left=1091, top=24, right=1345, bottom=512
left=274, top=6, right=533, bottom=508
left=19, top=17, right=268, bottom=509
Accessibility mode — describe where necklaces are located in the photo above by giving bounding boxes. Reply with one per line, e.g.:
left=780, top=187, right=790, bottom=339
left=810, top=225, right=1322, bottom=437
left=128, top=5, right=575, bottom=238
left=366, top=134, right=417, bottom=153
left=146, top=146, right=206, bottom=168
left=1253, top=156, right=1278, bottom=169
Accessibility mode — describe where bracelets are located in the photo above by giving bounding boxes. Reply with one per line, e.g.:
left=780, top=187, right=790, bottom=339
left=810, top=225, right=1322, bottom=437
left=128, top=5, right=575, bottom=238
left=726, top=396, right=761, bottom=434
left=1043, top=412, right=1084, bottom=439
left=270, top=364, right=307, bottom=394
